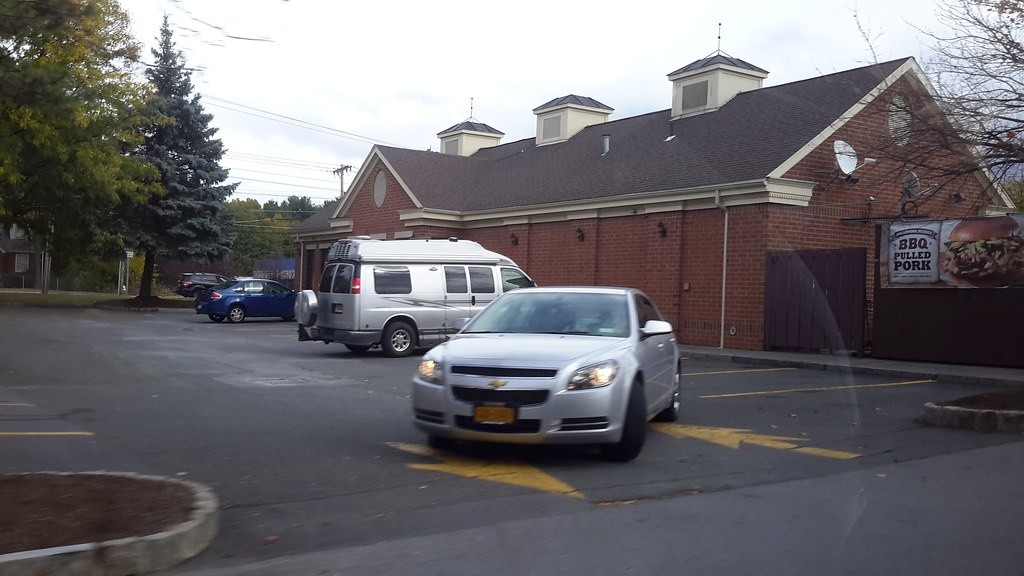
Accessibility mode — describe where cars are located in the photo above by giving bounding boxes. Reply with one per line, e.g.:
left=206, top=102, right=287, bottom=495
left=410, top=285, right=681, bottom=463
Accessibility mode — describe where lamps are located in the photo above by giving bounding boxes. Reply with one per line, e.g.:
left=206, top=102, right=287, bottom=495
left=510, top=233, right=518, bottom=246
left=576, top=228, right=585, bottom=242
left=843, top=175, right=860, bottom=185
left=657, top=222, right=668, bottom=238
left=953, top=192, right=966, bottom=203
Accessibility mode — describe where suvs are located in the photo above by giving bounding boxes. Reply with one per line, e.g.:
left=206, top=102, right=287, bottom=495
left=295, top=232, right=556, bottom=358
left=175, top=273, right=231, bottom=298
left=192, top=278, right=297, bottom=323
